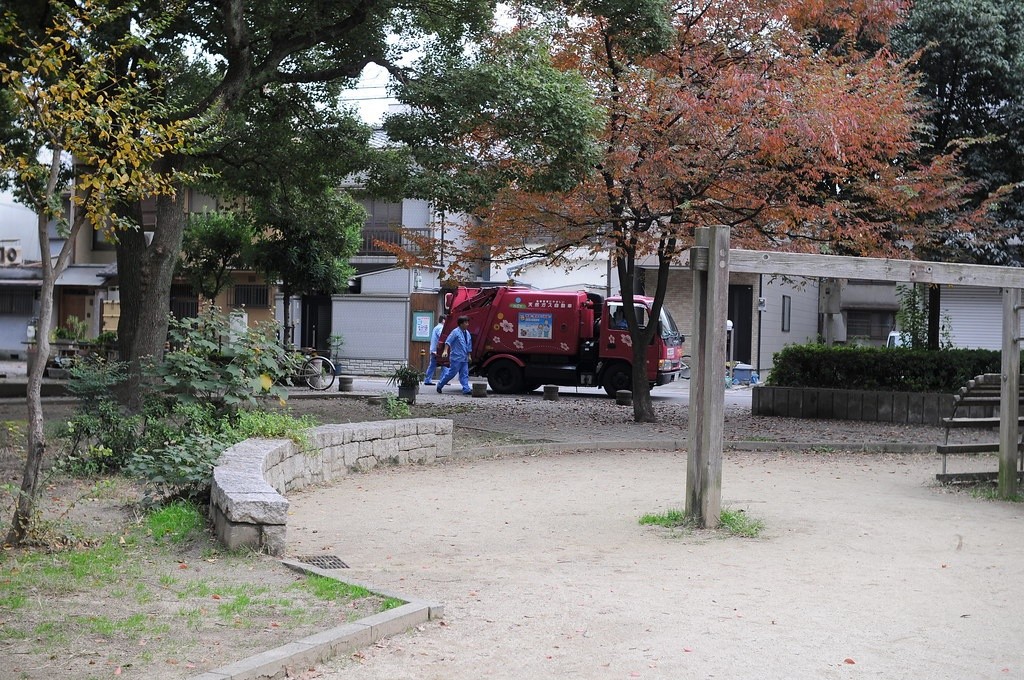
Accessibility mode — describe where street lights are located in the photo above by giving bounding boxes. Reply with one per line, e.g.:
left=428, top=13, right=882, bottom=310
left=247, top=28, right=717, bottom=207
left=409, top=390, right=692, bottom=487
left=726, top=319, right=734, bottom=384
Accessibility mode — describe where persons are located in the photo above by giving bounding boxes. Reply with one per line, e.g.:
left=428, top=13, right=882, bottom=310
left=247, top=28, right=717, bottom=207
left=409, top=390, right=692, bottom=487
left=437, top=317, right=473, bottom=394
left=617, top=312, right=628, bottom=329
left=424, top=314, right=451, bottom=385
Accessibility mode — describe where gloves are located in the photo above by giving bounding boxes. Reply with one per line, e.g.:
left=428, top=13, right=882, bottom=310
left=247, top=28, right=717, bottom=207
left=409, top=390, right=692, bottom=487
left=468, top=355, right=472, bottom=364
left=442, top=351, right=448, bottom=358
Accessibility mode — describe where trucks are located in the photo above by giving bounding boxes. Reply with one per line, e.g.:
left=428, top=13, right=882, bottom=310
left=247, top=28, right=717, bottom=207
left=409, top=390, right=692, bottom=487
left=431, top=281, right=687, bottom=398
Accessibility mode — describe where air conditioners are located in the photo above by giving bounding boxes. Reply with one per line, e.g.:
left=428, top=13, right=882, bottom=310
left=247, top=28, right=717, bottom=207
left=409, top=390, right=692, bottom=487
left=0, top=246, right=23, bottom=267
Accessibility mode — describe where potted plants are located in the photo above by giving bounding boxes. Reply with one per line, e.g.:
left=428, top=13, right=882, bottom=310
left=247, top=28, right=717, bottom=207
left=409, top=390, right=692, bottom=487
left=326, top=332, right=344, bottom=376
left=386, top=363, right=427, bottom=404
left=26, top=315, right=117, bottom=381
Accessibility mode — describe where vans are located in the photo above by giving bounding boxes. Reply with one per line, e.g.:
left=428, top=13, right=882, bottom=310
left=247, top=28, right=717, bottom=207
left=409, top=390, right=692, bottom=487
left=886, top=330, right=914, bottom=348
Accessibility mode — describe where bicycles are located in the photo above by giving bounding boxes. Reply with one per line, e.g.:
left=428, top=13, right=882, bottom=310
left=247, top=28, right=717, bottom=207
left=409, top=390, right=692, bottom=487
left=274, top=339, right=336, bottom=391
left=677, top=354, right=692, bottom=381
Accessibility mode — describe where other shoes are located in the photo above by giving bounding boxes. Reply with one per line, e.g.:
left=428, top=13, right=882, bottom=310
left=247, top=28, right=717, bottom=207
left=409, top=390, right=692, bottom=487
left=437, top=383, right=443, bottom=394
left=424, top=381, right=436, bottom=385
left=463, top=390, right=473, bottom=395
